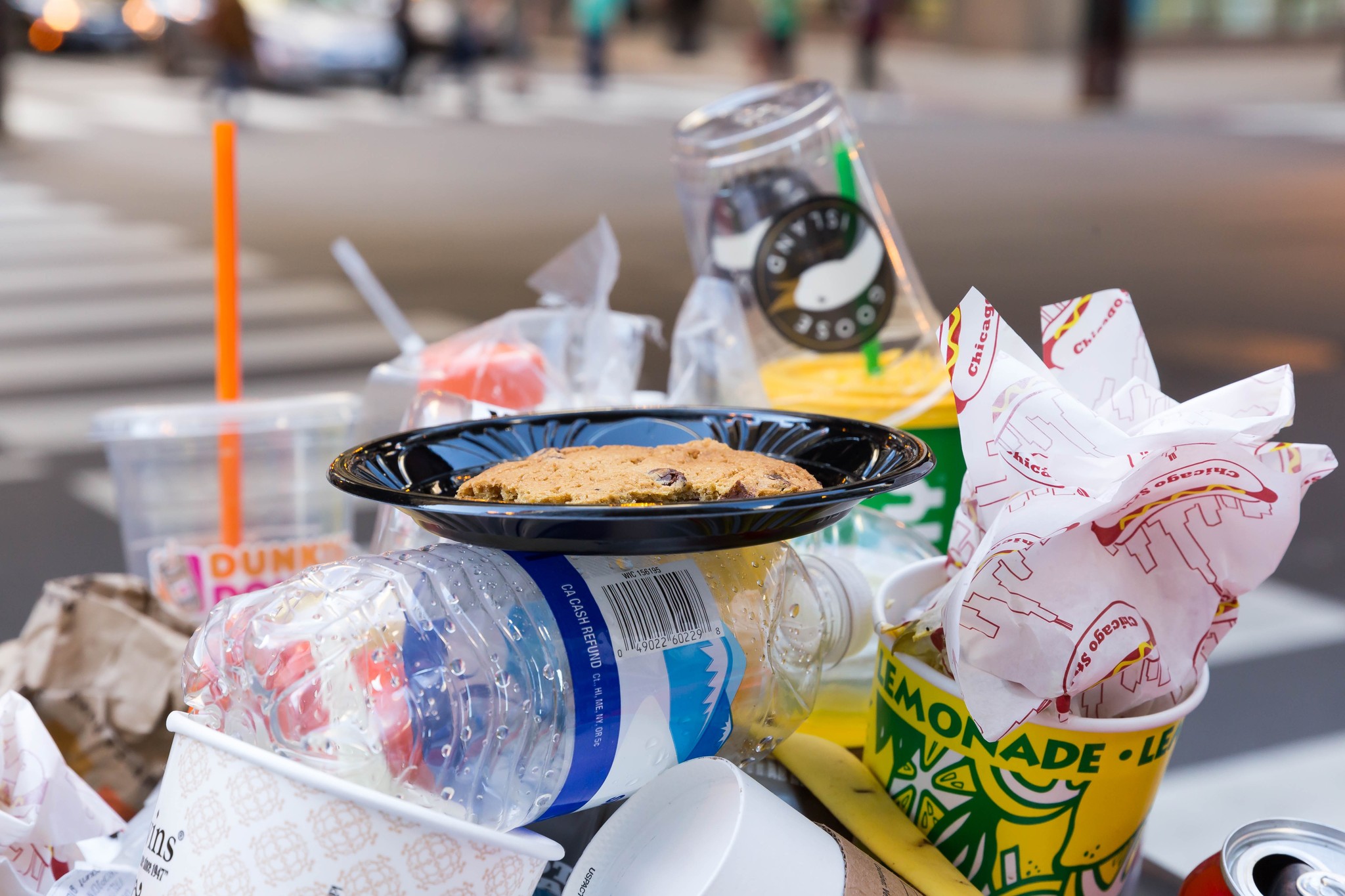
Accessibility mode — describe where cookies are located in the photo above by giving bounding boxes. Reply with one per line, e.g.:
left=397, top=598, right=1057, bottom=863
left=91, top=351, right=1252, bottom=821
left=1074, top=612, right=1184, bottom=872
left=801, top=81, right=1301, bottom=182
left=457, top=438, right=822, bottom=504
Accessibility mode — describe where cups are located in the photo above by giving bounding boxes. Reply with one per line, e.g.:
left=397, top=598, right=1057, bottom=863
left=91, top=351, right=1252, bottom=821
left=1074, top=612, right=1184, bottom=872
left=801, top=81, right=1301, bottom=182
left=665, top=79, right=948, bottom=428
left=876, top=556, right=1208, bottom=896
left=133, top=708, right=566, bottom=895
left=94, top=389, right=362, bottom=629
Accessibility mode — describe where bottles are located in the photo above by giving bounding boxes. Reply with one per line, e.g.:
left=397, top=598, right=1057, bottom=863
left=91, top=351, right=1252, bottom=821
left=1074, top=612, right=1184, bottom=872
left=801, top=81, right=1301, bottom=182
left=181, top=541, right=874, bottom=831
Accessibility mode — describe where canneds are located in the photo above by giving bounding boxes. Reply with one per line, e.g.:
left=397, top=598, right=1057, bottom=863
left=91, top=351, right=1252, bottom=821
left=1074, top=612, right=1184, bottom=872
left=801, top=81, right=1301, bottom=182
left=1176, top=818, right=1345, bottom=896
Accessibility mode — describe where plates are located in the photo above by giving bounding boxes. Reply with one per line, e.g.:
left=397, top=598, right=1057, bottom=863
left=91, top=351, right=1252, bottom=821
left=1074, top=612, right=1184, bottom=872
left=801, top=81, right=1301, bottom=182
left=327, top=403, right=936, bottom=549
